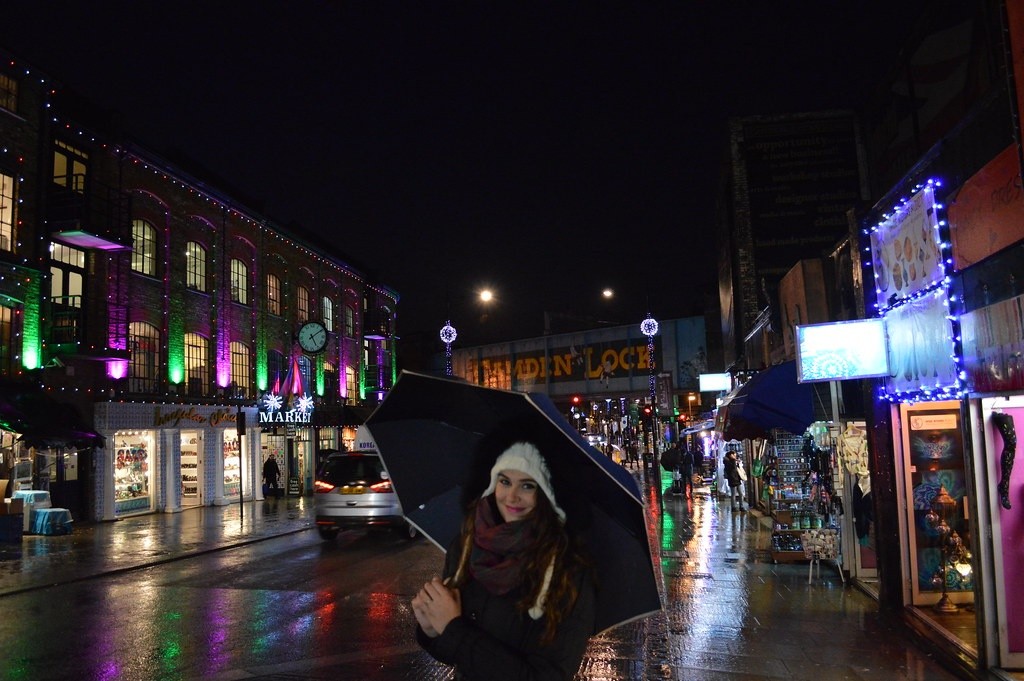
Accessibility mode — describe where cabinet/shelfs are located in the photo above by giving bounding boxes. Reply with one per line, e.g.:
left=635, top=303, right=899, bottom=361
left=770, top=426, right=835, bottom=565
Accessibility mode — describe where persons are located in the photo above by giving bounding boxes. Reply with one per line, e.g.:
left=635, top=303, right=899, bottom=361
left=671, top=440, right=718, bottom=499
left=600, top=360, right=615, bottom=388
left=262, top=454, right=281, bottom=500
left=723, top=450, right=747, bottom=512
left=0, top=452, right=15, bottom=498
left=412, top=440, right=597, bottom=681
left=596, top=443, right=641, bottom=469
left=570, top=346, right=583, bottom=367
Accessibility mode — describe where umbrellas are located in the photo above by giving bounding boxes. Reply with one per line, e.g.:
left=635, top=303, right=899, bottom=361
left=660, top=449, right=682, bottom=472
left=365, top=367, right=663, bottom=636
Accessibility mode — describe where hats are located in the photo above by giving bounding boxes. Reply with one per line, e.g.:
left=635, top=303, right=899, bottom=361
left=480, top=444, right=568, bottom=522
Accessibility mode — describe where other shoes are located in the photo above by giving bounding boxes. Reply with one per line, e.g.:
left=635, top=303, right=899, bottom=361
left=274, top=497, right=279, bottom=499
left=263, top=495, right=267, bottom=499
left=731, top=507, right=739, bottom=512
left=739, top=506, right=747, bottom=511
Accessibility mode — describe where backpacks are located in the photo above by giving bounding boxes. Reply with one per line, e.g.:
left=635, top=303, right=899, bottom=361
left=723, top=468, right=729, bottom=479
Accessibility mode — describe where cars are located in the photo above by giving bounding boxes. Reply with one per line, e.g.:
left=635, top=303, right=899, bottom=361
left=582, top=433, right=600, bottom=442
left=313, top=450, right=419, bottom=540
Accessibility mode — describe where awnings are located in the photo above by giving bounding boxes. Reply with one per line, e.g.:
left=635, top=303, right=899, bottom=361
left=0, top=398, right=107, bottom=454
left=715, top=360, right=815, bottom=444
left=681, top=421, right=715, bottom=435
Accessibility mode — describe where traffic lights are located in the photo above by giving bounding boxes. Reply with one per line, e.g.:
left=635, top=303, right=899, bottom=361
left=573, top=396, right=579, bottom=403
left=643, top=407, right=651, bottom=414
left=675, top=414, right=690, bottom=421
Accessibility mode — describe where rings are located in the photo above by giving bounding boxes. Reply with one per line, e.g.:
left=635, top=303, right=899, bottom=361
left=424, top=597, right=429, bottom=602
